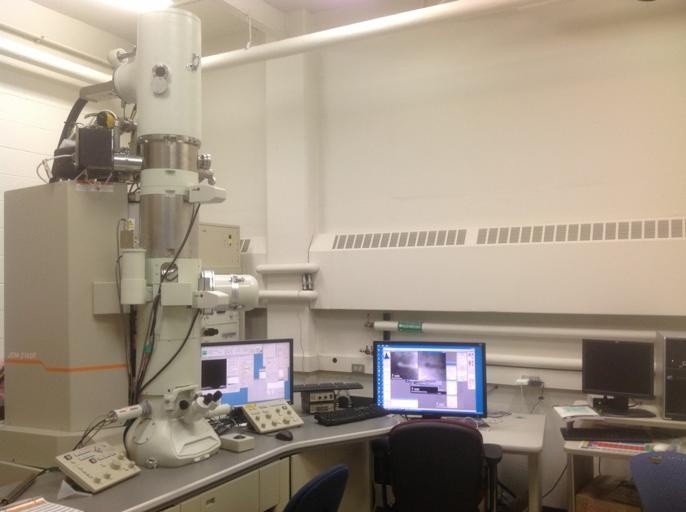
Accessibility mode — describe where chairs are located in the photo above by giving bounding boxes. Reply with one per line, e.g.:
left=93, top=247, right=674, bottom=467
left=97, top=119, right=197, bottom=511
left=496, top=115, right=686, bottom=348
left=373, top=416, right=505, bottom=511
left=261, top=462, right=353, bottom=511
left=630, top=449, right=686, bottom=512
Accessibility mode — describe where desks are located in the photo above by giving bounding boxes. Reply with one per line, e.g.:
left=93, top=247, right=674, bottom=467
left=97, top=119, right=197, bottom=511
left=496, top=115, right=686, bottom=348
left=563, top=406, right=685, bottom=512
left=0, top=404, right=547, bottom=512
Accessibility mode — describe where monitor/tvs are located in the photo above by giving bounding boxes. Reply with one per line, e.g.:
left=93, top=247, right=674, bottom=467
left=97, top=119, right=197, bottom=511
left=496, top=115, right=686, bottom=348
left=581, top=338, right=656, bottom=419
left=201, top=338, right=294, bottom=409
left=372, top=340, right=488, bottom=419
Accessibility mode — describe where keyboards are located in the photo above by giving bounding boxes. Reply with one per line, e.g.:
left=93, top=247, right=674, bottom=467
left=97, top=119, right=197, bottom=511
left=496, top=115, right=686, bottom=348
left=560, top=427, right=653, bottom=443
left=314, top=406, right=389, bottom=426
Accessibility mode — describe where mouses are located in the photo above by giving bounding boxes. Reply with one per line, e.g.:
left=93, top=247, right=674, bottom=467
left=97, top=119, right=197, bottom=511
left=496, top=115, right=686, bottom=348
left=276, top=430, right=293, bottom=441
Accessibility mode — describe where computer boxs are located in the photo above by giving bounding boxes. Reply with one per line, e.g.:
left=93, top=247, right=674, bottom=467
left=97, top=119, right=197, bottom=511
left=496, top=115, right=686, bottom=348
left=654, top=331, right=686, bottom=421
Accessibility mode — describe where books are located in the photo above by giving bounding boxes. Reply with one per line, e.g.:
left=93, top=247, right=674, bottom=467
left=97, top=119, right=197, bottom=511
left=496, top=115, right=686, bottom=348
left=553, top=403, right=600, bottom=421
